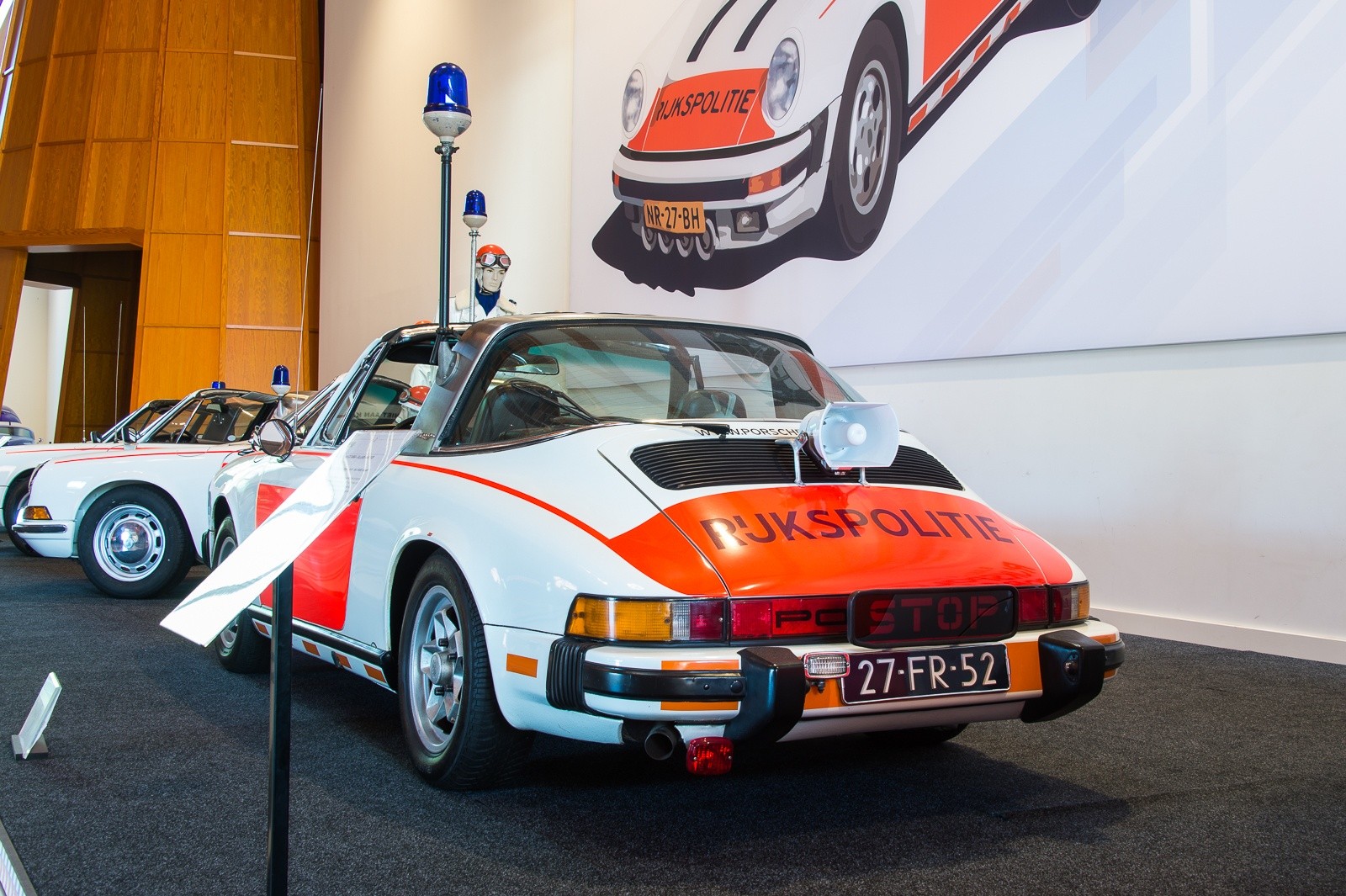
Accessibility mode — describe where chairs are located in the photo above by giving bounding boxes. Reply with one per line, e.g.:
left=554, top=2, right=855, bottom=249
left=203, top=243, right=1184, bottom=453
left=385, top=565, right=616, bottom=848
left=469, top=383, right=561, bottom=446
left=672, top=389, right=748, bottom=419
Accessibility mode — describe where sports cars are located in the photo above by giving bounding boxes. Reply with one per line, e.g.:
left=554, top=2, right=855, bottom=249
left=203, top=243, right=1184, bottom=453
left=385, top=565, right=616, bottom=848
left=199, top=304, right=1125, bottom=796
left=1, top=362, right=326, bottom=600
